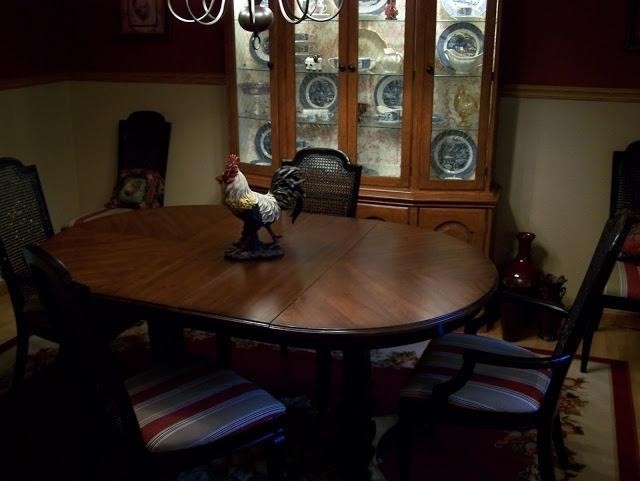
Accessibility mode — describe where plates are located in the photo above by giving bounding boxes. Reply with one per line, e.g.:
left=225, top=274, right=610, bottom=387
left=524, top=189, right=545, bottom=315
left=373, top=75, right=407, bottom=121
left=253, top=121, right=273, bottom=163
left=440, top=0, right=487, bottom=19
left=297, top=73, right=339, bottom=114
left=249, top=28, right=273, bottom=66
left=437, top=20, right=485, bottom=73
left=359, top=0, right=387, bottom=17
left=429, top=129, right=478, bottom=179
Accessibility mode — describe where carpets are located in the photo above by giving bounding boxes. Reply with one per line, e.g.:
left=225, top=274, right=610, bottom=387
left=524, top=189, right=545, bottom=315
left=1, top=319, right=640, bottom=479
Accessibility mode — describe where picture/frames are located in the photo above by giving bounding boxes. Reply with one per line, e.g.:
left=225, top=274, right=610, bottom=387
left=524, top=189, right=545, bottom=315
left=110, top=0, right=174, bottom=44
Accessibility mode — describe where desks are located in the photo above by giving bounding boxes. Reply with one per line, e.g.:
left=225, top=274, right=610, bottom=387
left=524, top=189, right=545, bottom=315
left=37, top=206, right=502, bottom=481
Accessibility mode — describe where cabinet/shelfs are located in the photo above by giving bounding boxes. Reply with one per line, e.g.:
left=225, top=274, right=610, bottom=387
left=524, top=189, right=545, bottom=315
left=225, top=0, right=503, bottom=258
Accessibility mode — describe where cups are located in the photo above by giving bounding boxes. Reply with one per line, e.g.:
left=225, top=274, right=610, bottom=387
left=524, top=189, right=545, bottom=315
left=357, top=56, right=376, bottom=73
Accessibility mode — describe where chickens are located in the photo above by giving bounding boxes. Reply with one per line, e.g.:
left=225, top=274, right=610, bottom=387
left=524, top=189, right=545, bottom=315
left=215, top=154, right=306, bottom=251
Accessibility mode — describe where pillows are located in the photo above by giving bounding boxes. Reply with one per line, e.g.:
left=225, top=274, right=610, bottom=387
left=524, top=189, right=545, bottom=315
left=105, top=169, right=159, bottom=209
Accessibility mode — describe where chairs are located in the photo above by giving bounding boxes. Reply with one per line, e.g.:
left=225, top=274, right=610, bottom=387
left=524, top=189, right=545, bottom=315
left=396, top=209, right=629, bottom=480
left=579, top=144, right=640, bottom=374
left=100, top=352, right=289, bottom=481
left=1, top=158, right=140, bottom=419
left=61, top=111, right=172, bottom=231
